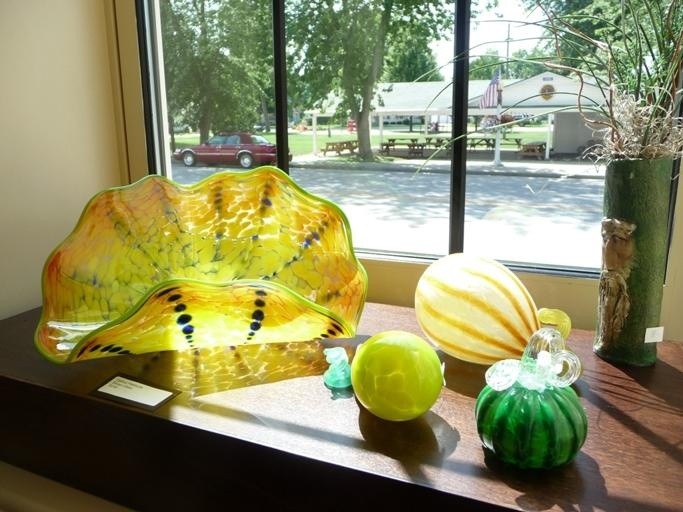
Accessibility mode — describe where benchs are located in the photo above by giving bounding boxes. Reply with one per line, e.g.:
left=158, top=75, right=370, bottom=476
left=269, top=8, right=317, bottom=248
left=320, top=137, right=555, bottom=161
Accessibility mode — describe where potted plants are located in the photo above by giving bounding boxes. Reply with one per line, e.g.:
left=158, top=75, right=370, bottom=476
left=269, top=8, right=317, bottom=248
left=396, top=0, right=683, bottom=369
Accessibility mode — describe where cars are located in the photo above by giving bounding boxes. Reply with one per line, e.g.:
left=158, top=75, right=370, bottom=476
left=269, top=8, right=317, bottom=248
left=174, top=132, right=292, bottom=168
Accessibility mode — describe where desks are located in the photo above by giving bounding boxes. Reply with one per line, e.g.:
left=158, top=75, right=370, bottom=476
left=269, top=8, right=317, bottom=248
left=0, top=294, right=680, bottom=512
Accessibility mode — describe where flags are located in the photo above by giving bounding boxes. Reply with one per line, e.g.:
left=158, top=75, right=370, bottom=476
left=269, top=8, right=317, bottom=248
left=479, top=66, right=499, bottom=110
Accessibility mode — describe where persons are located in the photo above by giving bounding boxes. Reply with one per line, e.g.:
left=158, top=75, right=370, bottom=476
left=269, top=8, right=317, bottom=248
left=478, top=114, right=498, bottom=148
left=426, top=121, right=439, bottom=133
